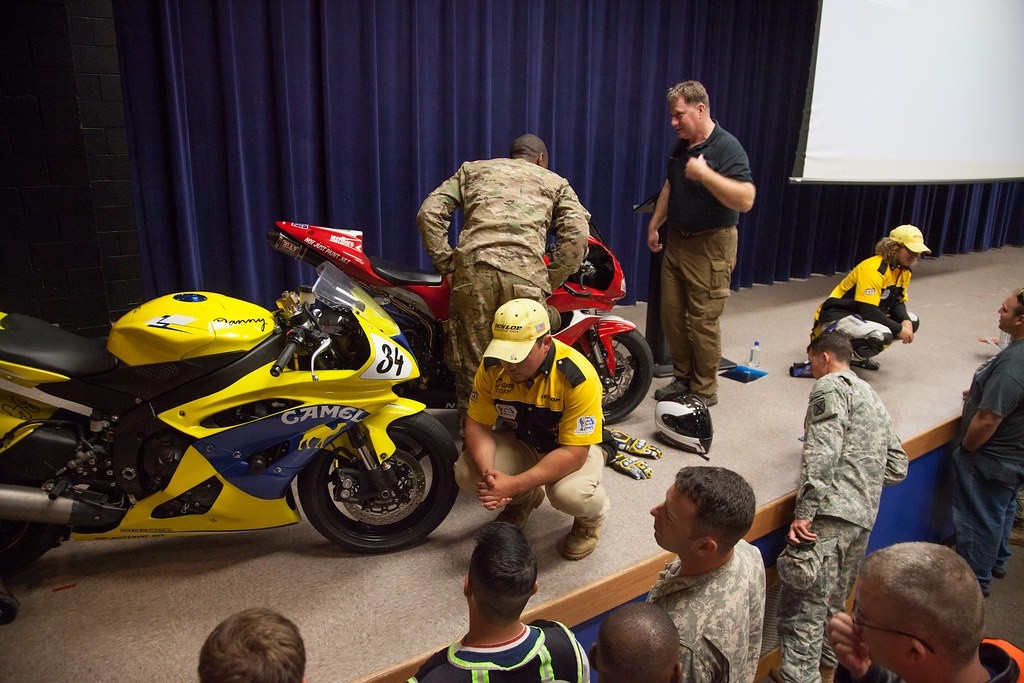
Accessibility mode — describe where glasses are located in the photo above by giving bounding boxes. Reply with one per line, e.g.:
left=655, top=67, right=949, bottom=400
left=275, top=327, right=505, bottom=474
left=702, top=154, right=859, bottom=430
left=850, top=597, right=937, bottom=655
left=1017, top=288, right=1024, bottom=303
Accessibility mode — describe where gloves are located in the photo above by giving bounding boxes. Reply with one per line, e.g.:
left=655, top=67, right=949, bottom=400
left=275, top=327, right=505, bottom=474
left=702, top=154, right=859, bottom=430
left=610, top=430, right=661, bottom=459
left=607, top=452, right=653, bottom=480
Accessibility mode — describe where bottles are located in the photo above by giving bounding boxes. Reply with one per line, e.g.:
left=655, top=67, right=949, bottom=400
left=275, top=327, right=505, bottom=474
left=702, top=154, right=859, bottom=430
left=999, top=327, right=1011, bottom=350
left=749, top=340, right=762, bottom=368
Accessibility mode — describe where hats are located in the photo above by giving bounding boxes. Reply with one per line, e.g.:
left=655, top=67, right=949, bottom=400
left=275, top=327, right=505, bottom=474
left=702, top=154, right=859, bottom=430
left=890, top=223, right=932, bottom=253
left=483, top=298, right=551, bottom=363
left=777, top=533, right=823, bottom=590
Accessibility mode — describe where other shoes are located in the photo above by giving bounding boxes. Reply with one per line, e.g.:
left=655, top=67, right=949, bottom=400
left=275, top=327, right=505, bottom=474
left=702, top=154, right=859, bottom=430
left=849, top=351, right=881, bottom=369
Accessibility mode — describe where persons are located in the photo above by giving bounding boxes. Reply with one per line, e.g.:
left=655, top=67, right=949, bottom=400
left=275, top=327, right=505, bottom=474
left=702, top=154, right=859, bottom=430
left=455, top=300, right=619, bottom=561
left=949, top=283, right=1024, bottom=597
left=589, top=601, right=685, bottom=683
left=807, top=224, right=932, bottom=370
left=195, top=608, right=307, bottom=683
left=824, top=540, right=1024, bottom=683
left=416, top=131, right=591, bottom=452
left=769, top=326, right=909, bottom=683
left=643, top=464, right=766, bottom=683
left=406, top=518, right=592, bottom=683
left=647, top=78, right=757, bottom=407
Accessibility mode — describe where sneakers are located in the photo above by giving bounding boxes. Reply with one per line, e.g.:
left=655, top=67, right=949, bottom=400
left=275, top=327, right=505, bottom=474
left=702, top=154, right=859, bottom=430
left=656, top=378, right=689, bottom=400
left=564, top=513, right=603, bottom=559
left=493, top=487, right=544, bottom=529
left=690, top=392, right=718, bottom=405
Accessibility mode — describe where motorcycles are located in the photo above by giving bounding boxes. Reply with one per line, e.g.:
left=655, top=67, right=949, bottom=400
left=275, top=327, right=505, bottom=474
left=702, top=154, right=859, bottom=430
left=0, top=261, right=460, bottom=574
left=268, top=222, right=654, bottom=424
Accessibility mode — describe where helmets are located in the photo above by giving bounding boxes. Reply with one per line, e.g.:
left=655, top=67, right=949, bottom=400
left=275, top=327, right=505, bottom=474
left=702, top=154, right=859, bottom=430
left=655, top=392, right=714, bottom=454
